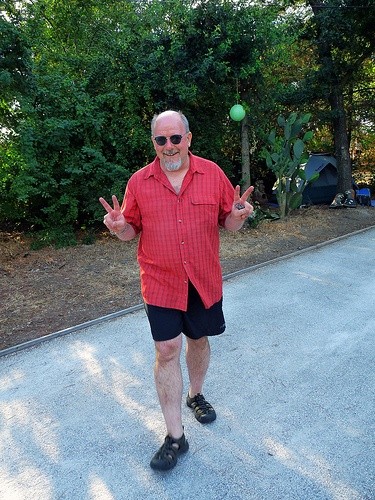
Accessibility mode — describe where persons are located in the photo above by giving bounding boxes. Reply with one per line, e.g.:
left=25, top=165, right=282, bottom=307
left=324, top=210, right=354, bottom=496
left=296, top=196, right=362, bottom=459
left=99, top=110, right=254, bottom=471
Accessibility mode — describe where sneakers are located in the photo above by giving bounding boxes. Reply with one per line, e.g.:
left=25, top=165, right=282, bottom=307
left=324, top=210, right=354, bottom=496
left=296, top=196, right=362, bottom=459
left=185, top=393, right=216, bottom=425
left=151, top=433, right=190, bottom=471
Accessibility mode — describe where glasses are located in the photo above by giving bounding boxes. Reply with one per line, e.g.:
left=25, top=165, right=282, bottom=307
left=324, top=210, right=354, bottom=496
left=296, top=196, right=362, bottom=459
left=150, top=132, right=187, bottom=146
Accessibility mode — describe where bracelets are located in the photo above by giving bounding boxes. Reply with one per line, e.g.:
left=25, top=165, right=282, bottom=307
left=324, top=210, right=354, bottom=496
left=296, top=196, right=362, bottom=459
left=109, top=222, right=128, bottom=235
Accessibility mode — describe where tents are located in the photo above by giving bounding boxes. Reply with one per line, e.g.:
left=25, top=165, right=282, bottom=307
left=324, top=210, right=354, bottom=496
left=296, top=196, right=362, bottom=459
left=272, top=151, right=360, bottom=206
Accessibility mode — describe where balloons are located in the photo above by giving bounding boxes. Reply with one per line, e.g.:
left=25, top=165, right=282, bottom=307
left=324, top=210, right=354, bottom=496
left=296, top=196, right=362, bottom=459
left=229, top=104, right=246, bottom=122
